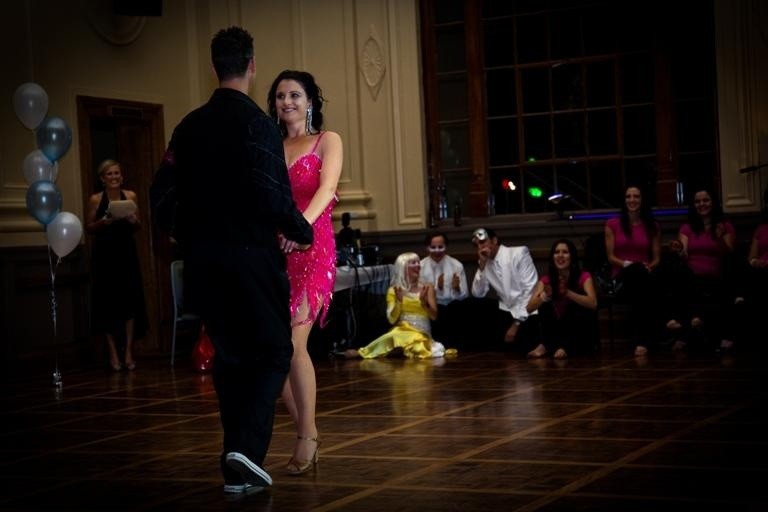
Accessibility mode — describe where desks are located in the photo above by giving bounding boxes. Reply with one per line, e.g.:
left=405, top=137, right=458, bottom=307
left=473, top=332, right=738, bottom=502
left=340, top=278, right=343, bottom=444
left=334, top=263, right=395, bottom=295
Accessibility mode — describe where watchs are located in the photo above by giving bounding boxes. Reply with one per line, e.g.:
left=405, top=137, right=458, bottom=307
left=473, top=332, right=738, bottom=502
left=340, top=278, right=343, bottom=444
left=513, top=320, right=521, bottom=325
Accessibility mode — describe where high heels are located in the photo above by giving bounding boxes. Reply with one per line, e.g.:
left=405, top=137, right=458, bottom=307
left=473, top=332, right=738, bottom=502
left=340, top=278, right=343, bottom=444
left=283, top=436, right=322, bottom=474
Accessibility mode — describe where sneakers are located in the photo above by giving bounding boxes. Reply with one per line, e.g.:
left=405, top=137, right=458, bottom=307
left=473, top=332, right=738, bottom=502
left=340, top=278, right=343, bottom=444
left=224, top=482, right=265, bottom=492
left=225, top=445, right=272, bottom=487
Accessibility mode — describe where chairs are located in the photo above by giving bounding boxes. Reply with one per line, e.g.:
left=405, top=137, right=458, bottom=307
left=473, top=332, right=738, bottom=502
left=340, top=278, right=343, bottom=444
left=170, top=260, right=197, bottom=365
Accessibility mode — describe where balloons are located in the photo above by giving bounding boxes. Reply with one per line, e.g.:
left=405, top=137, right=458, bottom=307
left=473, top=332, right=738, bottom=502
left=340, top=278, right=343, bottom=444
left=23, top=149, right=59, bottom=184
left=47, top=211, right=83, bottom=264
left=26, top=180, right=62, bottom=232
left=14, top=83, right=49, bottom=130
left=37, top=115, right=72, bottom=164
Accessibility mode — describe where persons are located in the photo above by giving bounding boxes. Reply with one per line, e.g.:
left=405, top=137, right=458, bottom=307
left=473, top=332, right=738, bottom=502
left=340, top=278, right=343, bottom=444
left=85, top=159, right=150, bottom=372
left=268, top=71, right=343, bottom=475
left=670, top=187, right=737, bottom=338
left=344, top=252, right=459, bottom=359
left=527, top=240, right=597, bottom=360
left=604, top=181, right=682, bottom=356
left=471, top=226, right=538, bottom=354
left=338, top=212, right=351, bottom=234
left=417, top=231, right=469, bottom=352
left=721, top=191, right=768, bottom=349
left=150, top=28, right=311, bottom=493
